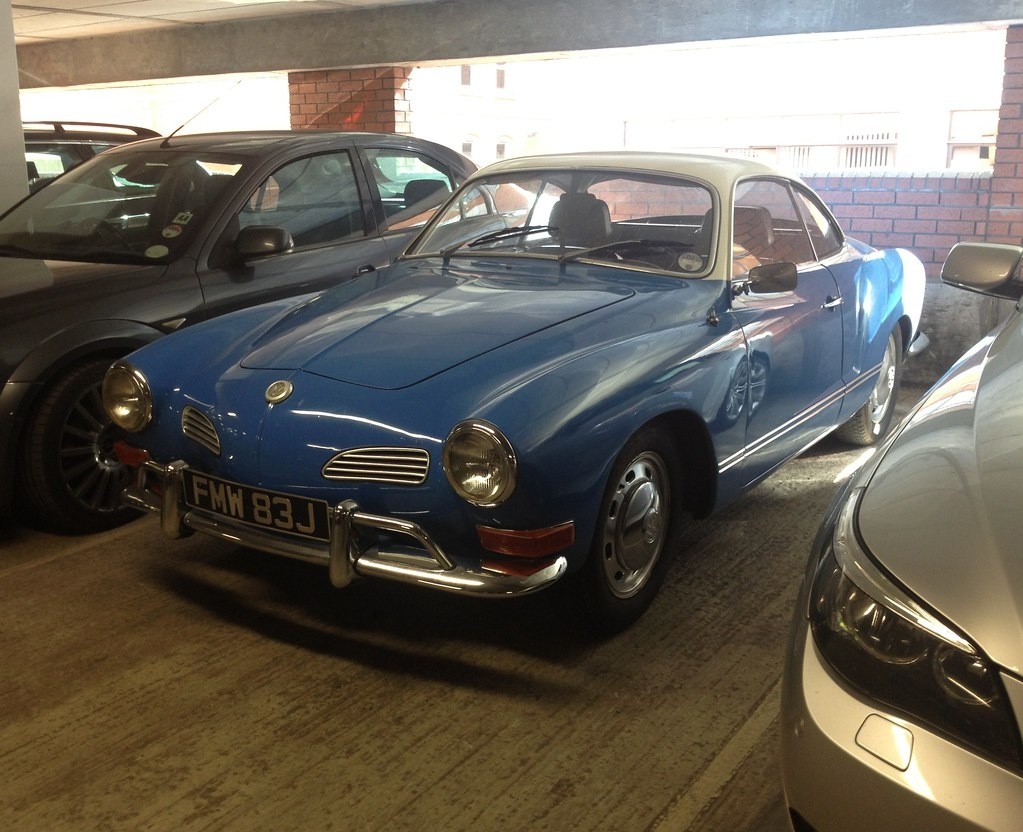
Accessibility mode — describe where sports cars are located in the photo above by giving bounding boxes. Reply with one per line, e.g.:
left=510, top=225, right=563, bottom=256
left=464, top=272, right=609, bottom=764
left=103, top=151, right=927, bottom=632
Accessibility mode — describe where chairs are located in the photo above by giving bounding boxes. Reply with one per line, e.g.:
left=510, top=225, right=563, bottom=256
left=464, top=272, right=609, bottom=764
left=547, top=192, right=612, bottom=245
left=281, top=157, right=353, bottom=247
left=173, top=174, right=258, bottom=270
left=404, top=179, right=446, bottom=208
left=698, top=207, right=776, bottom=266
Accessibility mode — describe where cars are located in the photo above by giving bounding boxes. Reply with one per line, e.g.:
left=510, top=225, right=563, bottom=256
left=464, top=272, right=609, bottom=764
left=782, top=241, right=1023, bottom=832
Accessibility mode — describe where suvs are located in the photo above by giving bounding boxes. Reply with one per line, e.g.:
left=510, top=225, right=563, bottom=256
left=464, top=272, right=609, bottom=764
left=22, top=121, right=213, bottom=253
left=0, top=129, right=481, bottom=537
